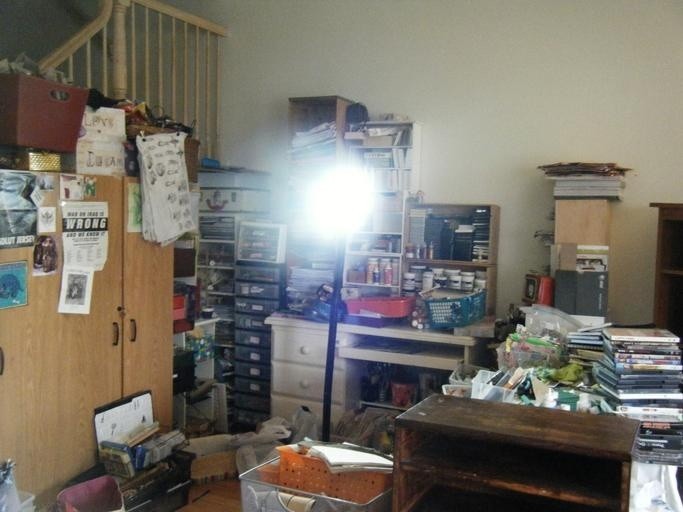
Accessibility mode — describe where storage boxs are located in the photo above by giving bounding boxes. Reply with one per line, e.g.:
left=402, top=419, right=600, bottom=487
left=554, top=268, right=610, bottom=318
left=287, top=95, right=356, bottom=152
left=607, top=199, right=660, bottom=328
left=0, top=73, right=91, bottom=152
left=183, top=138, right=202, bottom=184
left=548, top=243, right=609, bottom=270
left=238, top=455, right=396, bottom=512
left=236, top=221, right=288, bottom=264
left=197, top=186, right=271, bottom=214
left=196, top=170, right=274, bottom=191
left=276, top=443, right=394, bottom=503
left=422, top=289, right=486, bottom=330
left=10, top=151, right=62, bottom=172
left=63, top=448, right=196, bottom=512
left=552, top=198, right=607, bottom=244
left=345, top=297, right=416, bottom=318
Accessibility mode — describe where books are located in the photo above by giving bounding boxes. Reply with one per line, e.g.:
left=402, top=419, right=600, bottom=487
left=283, top=247, right=336, bottom=308
left=301, top=438, right=395, bottom=475
left=408, top=207, right=488, bottom=260
left=112, top=419, right=160, bottom=450
left=596, top=329, right=682, bottom=463
left=537, top=160, right=632, bottom=200
left=289, top=122, right=337, bottom=165
left=239, top=225, right=278, bottom=262
left=564, top=321, right=613, bottom=377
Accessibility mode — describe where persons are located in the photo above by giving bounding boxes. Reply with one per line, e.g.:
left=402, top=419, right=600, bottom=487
left=69, top=280, right=83, bottom=301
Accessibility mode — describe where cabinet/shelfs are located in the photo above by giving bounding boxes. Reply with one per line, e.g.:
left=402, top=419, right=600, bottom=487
left=648, top=202, right=683, bottom=343
left=393, top=392, right=644, bottom=512
left=230, top=260, right=286, bottom=436
left=173, top=315, right=222, bottom=440
left=339, top=120, right=422, bottom=308
left=193, top=212, right=255, bottom=431
left=0, top=170, right=175, bottom=512
left=400, top=202, right=501, bottom=320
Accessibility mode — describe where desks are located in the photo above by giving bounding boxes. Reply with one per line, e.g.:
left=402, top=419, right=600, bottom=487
left=265, top=311, right=485, bottom=434
